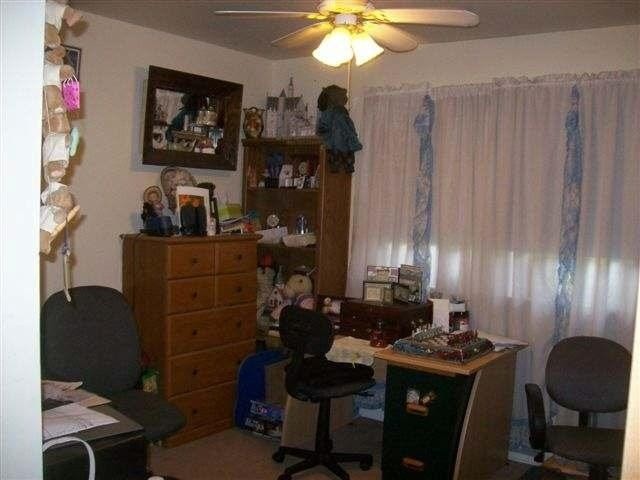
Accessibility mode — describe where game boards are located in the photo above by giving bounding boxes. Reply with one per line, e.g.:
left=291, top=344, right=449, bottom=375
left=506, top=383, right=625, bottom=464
left=393, top=334, right=495, bottom=365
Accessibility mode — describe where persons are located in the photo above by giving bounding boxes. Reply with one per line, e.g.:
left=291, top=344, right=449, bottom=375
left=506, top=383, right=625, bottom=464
left=181, top=197, right=207, bottom=228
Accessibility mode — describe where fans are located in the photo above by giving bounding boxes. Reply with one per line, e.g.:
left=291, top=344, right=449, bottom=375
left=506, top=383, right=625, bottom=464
left=213, top=0, right=481, bottom=53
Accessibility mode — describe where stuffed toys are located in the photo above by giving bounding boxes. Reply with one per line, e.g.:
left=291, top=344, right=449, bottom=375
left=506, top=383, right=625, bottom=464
left=257, top=253, right=314, bottom=331
left=40, top=0, right=85, bottom=257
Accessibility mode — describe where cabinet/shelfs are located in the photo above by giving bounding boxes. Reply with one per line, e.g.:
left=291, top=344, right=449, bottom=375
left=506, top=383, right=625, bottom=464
left=41, top=380, right=148, bottom=480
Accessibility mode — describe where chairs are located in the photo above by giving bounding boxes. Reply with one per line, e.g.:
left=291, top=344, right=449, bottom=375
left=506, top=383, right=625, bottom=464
left=41, top=284, right=188, bottom=480
left=523, top=335, right=632, bottom=480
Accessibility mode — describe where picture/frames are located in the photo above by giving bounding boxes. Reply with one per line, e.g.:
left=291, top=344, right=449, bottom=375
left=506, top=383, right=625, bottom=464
left=142, top=63, right=244, bottom=171
left=61, top=43, right=81, bottom=82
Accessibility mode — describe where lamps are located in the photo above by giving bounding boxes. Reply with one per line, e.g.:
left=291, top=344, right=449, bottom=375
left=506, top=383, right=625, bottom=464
left=313, top=28, right=385, bottom=68
